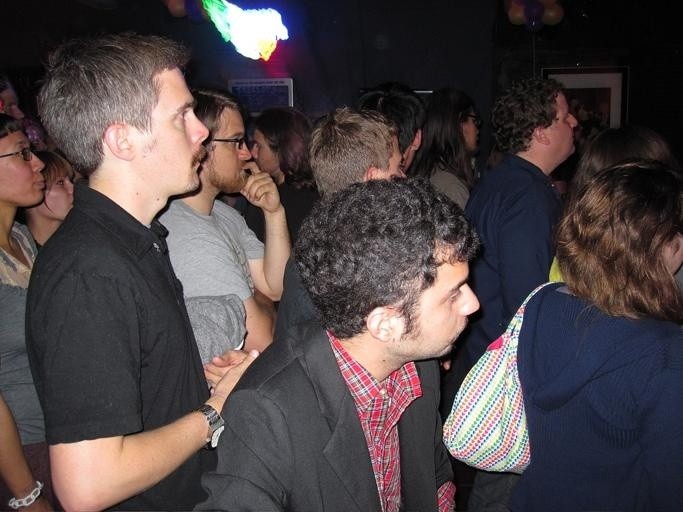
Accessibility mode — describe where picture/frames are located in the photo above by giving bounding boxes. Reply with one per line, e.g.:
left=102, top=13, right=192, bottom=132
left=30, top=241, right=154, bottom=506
left=535, top=63, right=630, bottom=182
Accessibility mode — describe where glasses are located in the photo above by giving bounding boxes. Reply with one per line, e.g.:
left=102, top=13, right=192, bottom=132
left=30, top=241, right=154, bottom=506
left=0, top=147, right=32, bottom=161
left=213, top=137, right=244, bottom=149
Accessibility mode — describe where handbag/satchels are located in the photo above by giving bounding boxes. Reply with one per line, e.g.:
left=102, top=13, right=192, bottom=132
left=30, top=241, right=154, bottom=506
left=442, top=282, right=557, bottom=475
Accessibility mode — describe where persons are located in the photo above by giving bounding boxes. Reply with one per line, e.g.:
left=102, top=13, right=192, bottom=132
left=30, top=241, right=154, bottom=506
left=172, top=95, right=291, bottom=361
left=464, top=163, right=683, bottom=511
left=0, top=69, right=576, bottom=321
left=271, top=104, right=396, bottom=349
left=441, top=79, right=576, bottom=501
left=549, top=125, right=666, bottom=299
left=27, top=36, right=265, bottom=511
left=0, top=116, right=53, bottom=511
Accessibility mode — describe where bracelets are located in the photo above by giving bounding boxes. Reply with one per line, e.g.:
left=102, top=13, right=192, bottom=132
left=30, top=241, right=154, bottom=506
left=7, top=478, right=43, bottom=509
left=200, top=172, right=484, bottom=510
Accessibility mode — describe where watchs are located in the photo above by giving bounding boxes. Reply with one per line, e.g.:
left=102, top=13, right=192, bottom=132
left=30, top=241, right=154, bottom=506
left=197, top=395, right=225, bottom=450
